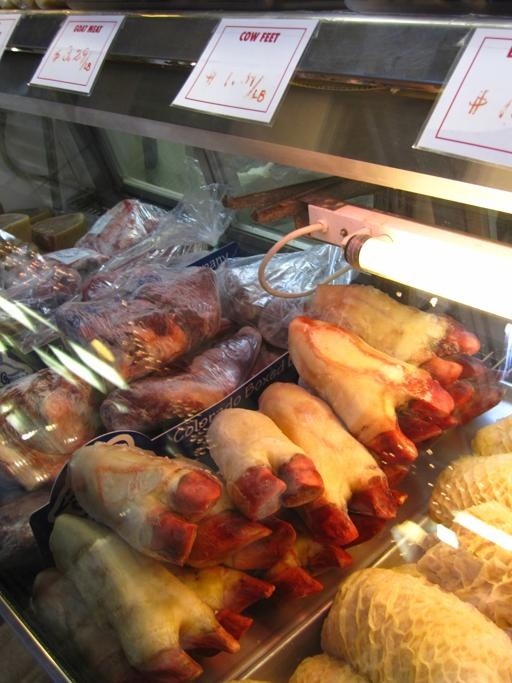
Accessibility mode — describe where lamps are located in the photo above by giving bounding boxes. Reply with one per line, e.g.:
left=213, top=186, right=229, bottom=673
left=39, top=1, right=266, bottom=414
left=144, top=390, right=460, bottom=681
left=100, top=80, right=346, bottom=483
left=306, top=204, right=512, bottom=324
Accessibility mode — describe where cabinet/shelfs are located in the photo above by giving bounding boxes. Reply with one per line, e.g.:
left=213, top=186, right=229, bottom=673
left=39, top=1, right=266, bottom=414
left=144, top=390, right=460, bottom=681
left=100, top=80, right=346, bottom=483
left=0, top=9, right=511, bottom=683
